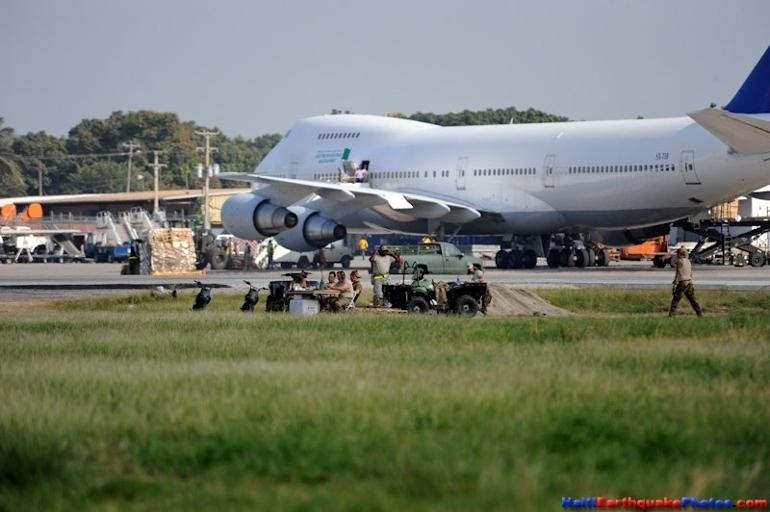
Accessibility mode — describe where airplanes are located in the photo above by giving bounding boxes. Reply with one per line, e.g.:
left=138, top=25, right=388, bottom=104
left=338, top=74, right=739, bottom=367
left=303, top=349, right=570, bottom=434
left=219, top=45, right=769, bottom=269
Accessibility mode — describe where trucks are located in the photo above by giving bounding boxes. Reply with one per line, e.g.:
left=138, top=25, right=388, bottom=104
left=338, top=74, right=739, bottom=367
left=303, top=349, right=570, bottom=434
left=95, top=242, right=131, bottom=264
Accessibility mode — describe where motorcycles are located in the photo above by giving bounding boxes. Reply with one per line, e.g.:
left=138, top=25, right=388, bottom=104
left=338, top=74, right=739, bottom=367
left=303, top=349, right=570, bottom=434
left=192, top=280, right=219, bottom=309
left=240, top=280, right=268, bottom=312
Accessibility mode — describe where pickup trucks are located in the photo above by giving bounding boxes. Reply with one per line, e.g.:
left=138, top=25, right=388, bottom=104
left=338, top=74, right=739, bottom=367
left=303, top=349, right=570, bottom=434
left=373, top=242, right=483, bottom=274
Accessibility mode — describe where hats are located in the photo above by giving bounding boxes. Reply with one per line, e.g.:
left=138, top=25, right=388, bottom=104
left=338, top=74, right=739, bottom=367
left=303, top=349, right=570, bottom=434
left=351, top=271, right=361, bottom=278
left=380, top=245, right=388, bottom=250
left=467, top=263, right=473, bottom=270
left=678, top=247, right=685, bottom=255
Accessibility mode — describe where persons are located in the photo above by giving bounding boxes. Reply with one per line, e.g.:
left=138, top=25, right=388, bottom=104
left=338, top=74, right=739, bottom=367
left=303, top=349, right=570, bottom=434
left=370, top=244, right=400, bottom=308
left=225, top=237, right=241, bottom=257
left=354, top=164, right=368, bottom=182
left=412, top=273, right=437, bottom=301
left=265, top=240, right=274, bottom=270
left=287, top=271, right=363, bottom=314
left=357, top=236, right=368, bottom=260
left=464, top=266, right=484, bottom=283
left=668, top=245, right=704, bottom=318
left=242, top=242, right=250, bottom=272
